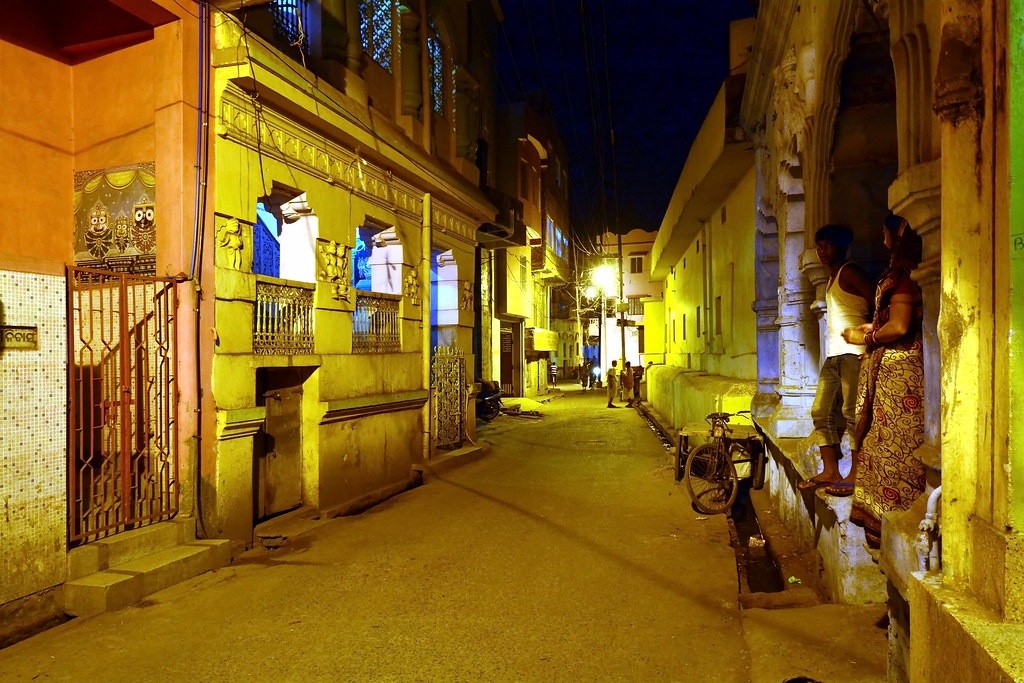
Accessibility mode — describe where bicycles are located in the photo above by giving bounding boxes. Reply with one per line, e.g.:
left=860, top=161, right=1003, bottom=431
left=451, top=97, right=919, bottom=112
left=684, top=410, right=751, bottom=515
left=552, top=376, right=555, bottom=388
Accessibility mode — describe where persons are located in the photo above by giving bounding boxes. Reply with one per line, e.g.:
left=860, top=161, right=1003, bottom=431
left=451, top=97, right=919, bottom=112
left=621, top=361, right=635, bottom=408
left=588, top=361, right=596, bottom=388
left=226, top=218, right=242, bottom=270
left=336, top=244, right=347, bottom=278
left=409, top=267, right=420, bottom=298
left=549, top=362, right=558, bottom=386
left=840, top=214, right=924, bottom=564
left=606, top=360, right=618, bottom=408
left=575, top=363, right=588, bottom=391
left=796, top=221, right=875, bottom=498
left=322, top=239, right=337, bottom=276
left=463, top=281, right=472, bottom=311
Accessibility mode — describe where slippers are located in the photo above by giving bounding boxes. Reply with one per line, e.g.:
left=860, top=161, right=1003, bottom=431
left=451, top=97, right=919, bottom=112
left=824, top=481, right=854, bottom=496
left=798, top=476, right=837, bottom=490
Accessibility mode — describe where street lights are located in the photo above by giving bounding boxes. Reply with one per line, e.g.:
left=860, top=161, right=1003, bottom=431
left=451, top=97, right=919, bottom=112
left=597, top=267, right=624, bottom=369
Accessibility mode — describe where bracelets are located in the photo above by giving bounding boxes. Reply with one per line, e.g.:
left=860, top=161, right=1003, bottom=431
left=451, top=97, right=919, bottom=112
left=863, top=330, right=879, bottom=346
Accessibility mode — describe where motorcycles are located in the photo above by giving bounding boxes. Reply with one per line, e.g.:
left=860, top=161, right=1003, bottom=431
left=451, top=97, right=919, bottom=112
left=476, top=379, right=505, bottom=421
